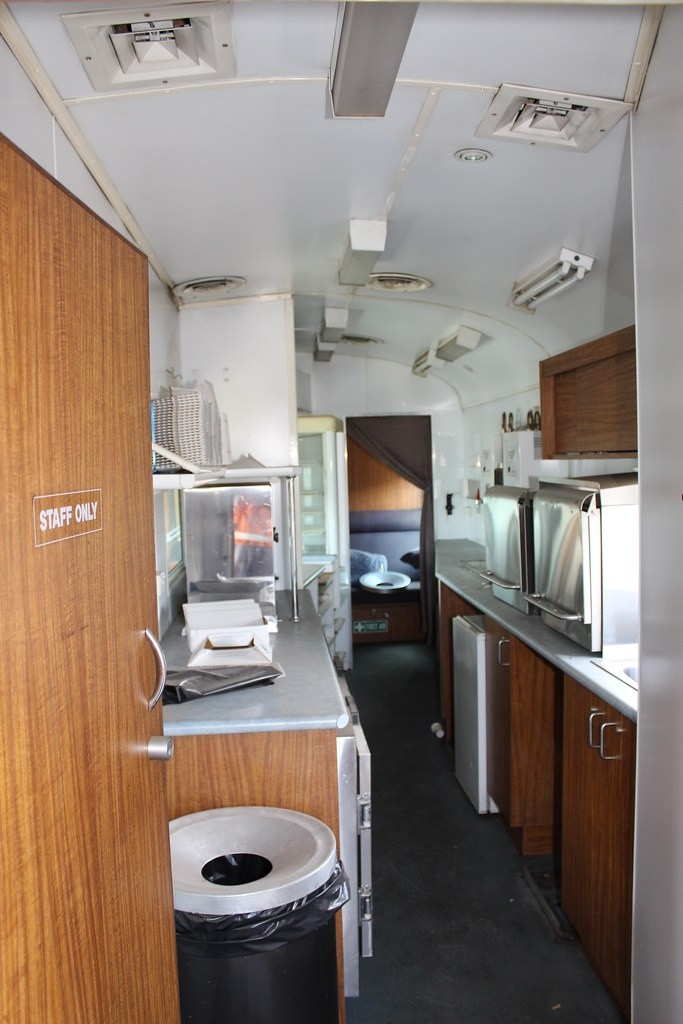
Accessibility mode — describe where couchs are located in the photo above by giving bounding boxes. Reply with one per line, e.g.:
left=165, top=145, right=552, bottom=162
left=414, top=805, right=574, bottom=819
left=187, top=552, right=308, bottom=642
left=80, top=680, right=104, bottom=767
left=350, top=510, right=427, bottom=643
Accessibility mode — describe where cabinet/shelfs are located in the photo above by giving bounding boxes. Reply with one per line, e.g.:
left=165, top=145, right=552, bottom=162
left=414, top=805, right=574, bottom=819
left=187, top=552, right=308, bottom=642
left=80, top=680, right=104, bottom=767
left=539, top=323, right=638, bottom=461
left=484, top=615, right=637, bottom=1024
left=167, top=415, right=372, bottom=998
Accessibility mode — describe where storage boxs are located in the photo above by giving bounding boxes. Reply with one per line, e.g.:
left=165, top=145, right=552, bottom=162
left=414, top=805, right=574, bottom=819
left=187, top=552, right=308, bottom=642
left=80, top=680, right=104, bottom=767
left=149, top=392, right=222, bottom=473
left=181, top=599, right=272, bottom=667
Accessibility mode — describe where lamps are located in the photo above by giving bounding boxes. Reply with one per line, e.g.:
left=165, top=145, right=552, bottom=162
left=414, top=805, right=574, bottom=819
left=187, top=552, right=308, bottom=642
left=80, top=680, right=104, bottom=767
left=508, top=247, right=594, bottom=315
left=411, top=350, right=442, bottom=379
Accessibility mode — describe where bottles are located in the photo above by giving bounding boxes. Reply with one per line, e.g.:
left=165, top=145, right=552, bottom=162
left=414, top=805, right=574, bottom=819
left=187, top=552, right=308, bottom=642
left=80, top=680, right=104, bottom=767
left=527, top=405, right=542, bottom=431
left=494, top=462, right=504, bottom=486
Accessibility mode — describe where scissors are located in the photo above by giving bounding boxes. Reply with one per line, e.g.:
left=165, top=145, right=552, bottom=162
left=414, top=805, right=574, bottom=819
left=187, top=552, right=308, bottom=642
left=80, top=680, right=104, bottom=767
left=527, top=411, right=541, bottom=430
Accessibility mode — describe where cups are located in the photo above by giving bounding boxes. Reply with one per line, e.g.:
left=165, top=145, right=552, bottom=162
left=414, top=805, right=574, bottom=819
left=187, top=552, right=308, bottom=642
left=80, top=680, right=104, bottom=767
left=431, top=722, right=444, bottom=737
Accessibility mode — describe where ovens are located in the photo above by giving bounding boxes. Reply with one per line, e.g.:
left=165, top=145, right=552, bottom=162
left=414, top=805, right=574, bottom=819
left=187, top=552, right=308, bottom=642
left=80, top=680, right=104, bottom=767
left=461, top=471, right=640, bottom=654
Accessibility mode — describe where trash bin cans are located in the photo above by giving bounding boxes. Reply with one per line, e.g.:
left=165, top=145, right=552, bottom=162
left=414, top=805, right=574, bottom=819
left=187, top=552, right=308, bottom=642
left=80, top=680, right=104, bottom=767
left=168, top=806, right=340, bottom=1024
